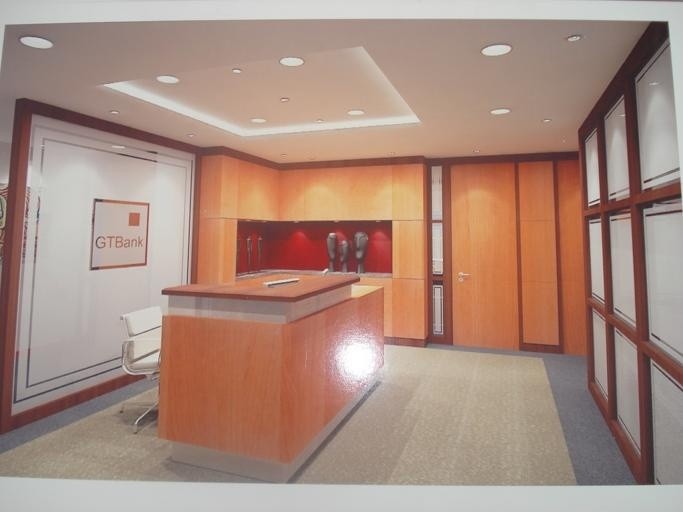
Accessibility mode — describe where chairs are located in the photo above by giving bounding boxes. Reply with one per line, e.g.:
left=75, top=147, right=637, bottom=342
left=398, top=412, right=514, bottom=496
left=119, top=305, right=162, bottom=433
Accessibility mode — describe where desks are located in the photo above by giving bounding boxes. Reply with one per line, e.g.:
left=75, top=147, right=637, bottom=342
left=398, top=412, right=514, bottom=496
left=159, top=272, right=384, bottom=483
